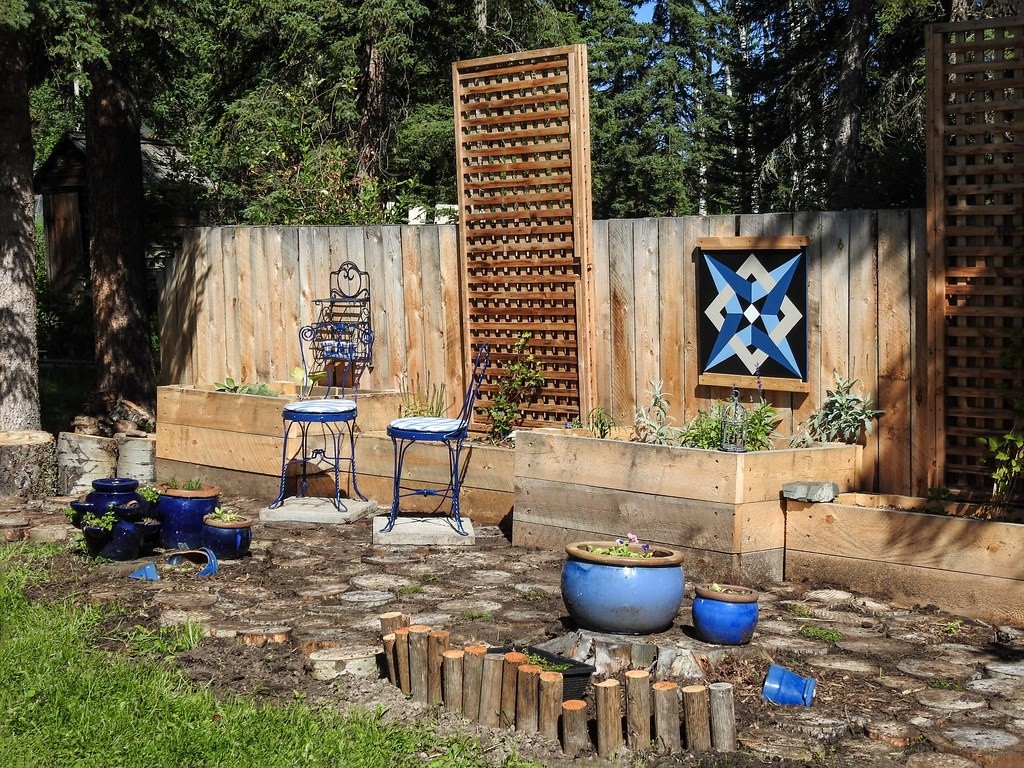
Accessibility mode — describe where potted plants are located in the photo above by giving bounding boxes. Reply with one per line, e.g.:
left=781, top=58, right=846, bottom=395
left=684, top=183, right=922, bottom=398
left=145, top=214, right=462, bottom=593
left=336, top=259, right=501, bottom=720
left=349, top=333, right=545, bottom=528
left=512, top=364, right=887, bottom=586
left=202, top=507, right=253, bottom=560
left=785, top=408, right=1024, bottom=628
left=156, top=362, right=405, bottom=498
left=71, top=502, right=159, bottom=560
left=693, top=581, right=760, bottom=646
left=154, top=473, right=222, bottom=548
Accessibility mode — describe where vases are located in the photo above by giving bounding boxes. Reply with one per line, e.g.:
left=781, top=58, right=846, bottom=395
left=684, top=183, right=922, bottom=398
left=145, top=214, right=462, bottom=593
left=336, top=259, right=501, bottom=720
left=87, top=477, right=148, bottom=518
left=561, top=542, right=684, bottom=634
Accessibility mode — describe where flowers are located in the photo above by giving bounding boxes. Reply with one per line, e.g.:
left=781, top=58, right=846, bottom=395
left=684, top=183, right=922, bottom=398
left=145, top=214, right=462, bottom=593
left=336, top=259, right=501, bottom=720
left=586, top=532, right=656, bottom=561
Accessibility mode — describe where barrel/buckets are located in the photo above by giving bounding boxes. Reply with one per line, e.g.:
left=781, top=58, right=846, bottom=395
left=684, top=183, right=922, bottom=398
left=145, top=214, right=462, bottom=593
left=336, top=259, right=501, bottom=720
left=762, top=663, right=816, bottom=708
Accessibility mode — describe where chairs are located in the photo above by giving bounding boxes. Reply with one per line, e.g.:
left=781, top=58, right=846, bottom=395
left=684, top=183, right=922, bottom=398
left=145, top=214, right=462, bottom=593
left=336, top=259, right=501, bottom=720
left=268, top=322, right=373, bottom=513
left=379, top=341, right=491, bottom=535
